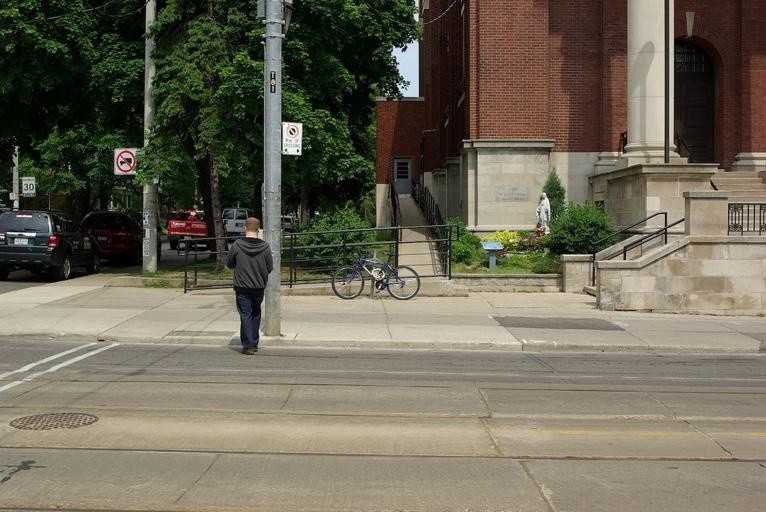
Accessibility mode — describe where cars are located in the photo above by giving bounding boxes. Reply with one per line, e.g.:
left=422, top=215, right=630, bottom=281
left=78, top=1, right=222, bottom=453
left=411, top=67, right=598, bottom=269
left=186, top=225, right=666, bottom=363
left=0, top=209, right=161, bottom=280
left=281, top=216, right=294, bottom=228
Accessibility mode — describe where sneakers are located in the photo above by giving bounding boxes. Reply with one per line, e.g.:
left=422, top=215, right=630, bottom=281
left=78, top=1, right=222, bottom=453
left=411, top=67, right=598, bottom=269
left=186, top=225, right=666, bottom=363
left=241, top=346, right=258, bottom=356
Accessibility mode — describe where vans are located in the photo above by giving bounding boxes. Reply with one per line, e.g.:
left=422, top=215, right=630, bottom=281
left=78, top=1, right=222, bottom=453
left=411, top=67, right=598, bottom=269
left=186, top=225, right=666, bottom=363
left=222, top=209, right=248, bottom=236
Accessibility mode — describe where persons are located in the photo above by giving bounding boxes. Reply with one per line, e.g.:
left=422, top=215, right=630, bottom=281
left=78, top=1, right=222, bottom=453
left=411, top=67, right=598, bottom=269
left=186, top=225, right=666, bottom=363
left=225, top=216, right=276, bottom=356
left=223, top=219, right=228, bottom=250
left=534, top=192, right=551, bottom=228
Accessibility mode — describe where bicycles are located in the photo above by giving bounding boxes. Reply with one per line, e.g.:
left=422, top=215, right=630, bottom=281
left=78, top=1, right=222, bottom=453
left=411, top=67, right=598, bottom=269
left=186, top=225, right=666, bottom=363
left=332, top=239, right=420, bottom=300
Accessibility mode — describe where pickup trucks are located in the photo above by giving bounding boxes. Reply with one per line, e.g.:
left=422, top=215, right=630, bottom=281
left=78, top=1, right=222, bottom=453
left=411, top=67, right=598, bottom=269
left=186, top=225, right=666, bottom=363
left=168, top=209, right=228, bottom=249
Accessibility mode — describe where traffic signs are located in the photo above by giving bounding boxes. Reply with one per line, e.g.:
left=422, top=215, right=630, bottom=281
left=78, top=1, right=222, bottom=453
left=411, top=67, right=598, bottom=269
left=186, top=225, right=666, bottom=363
left=22, top=177, right=36, bottom=197
